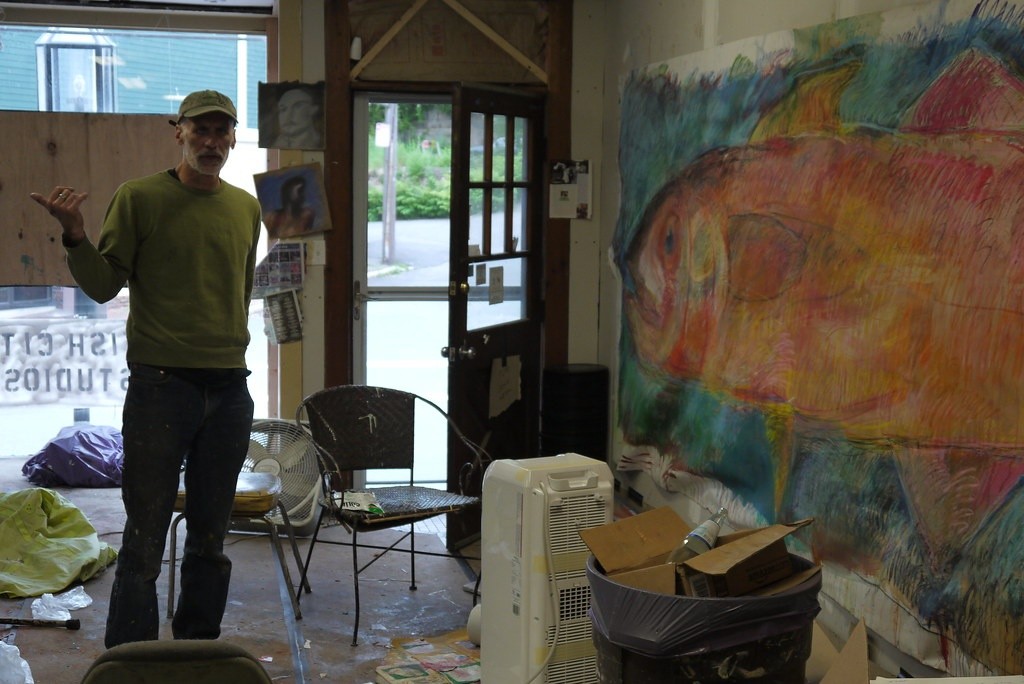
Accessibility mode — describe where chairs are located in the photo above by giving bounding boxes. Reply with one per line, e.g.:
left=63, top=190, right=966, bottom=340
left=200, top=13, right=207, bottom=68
left=296, top=384, right=486, bottom=644
left=81, top=640, right=273, bottom=684
left=168, top=499, right=312, bottom=623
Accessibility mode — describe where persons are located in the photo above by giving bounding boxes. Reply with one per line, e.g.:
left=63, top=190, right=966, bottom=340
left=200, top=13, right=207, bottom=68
left=28, top=90, right=263, bottom=649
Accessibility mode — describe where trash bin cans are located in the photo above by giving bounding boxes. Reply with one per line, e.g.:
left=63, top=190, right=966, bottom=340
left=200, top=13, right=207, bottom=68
left=585, top=551, right=823, bottom=684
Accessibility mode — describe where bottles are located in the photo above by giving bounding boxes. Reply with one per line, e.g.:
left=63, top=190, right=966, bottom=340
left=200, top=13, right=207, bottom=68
left=666, top=508, right=728, bottom=569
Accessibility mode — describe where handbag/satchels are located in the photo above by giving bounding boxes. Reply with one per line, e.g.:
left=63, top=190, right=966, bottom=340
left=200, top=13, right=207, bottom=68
left=21, top=423, right=124, bottom=489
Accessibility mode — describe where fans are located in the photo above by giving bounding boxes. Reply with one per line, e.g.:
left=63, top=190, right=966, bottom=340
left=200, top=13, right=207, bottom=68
left=230, top=417, right=325, bottom=539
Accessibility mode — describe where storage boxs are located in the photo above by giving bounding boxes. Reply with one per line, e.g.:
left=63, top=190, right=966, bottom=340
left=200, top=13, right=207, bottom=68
left=577, top=505, right=816, bottom=594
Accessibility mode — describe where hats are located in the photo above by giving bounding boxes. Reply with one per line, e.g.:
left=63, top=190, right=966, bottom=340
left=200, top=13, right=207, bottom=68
left=168, top=89, right=239, bottom=126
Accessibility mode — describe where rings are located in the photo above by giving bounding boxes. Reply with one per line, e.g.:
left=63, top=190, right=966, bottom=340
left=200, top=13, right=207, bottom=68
left=58, top=192, right=65, bottom=199
